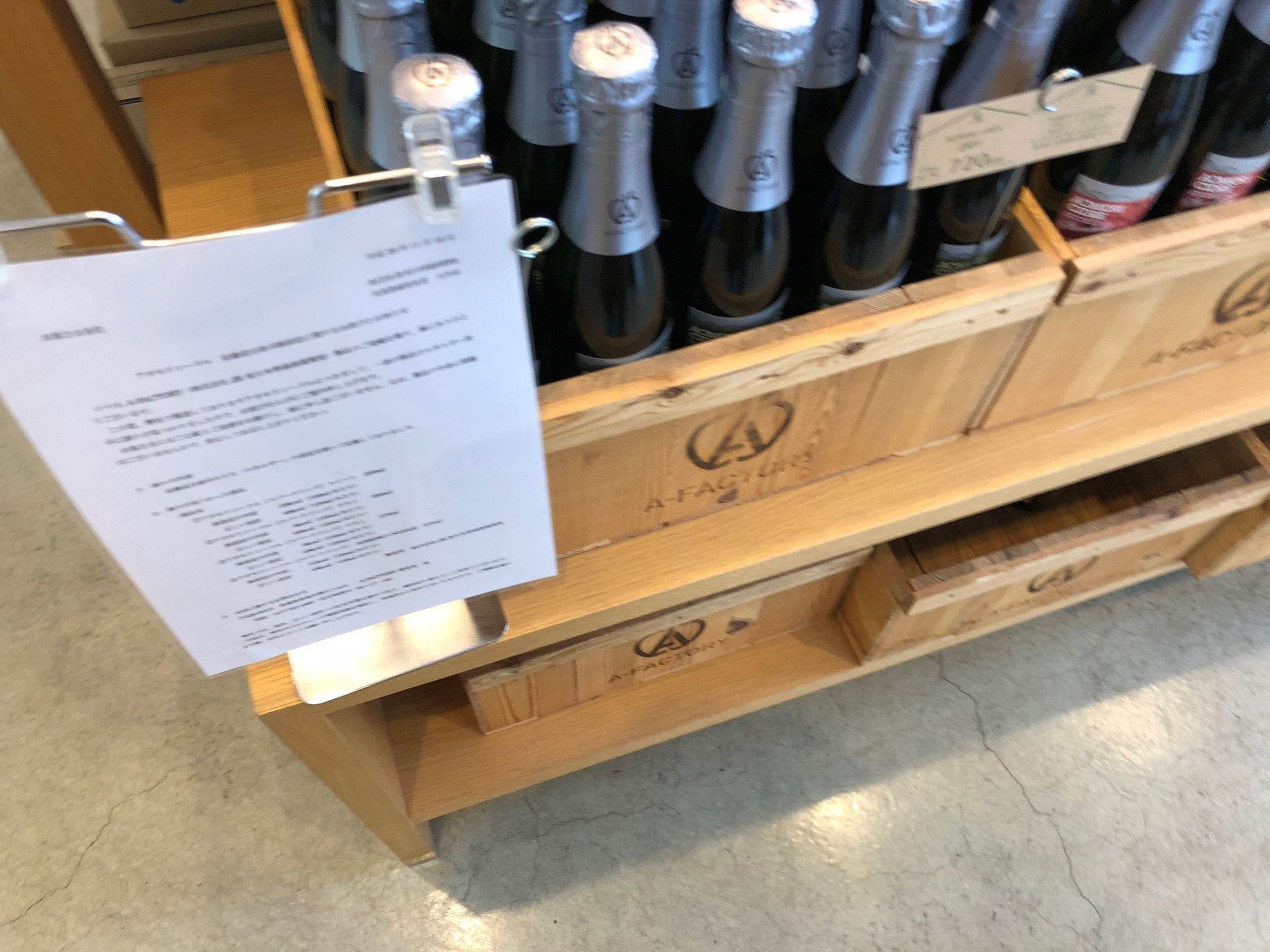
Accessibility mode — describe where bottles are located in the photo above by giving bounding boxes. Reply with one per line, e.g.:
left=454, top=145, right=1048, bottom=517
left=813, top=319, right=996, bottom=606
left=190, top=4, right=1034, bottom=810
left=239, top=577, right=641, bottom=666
left=294, top=0, right=1268, bottom=386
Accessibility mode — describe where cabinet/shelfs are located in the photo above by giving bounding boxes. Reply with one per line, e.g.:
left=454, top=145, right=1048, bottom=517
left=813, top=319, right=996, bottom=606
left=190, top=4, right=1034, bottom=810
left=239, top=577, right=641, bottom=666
left=142, top=0, right=1270, bottom=862
